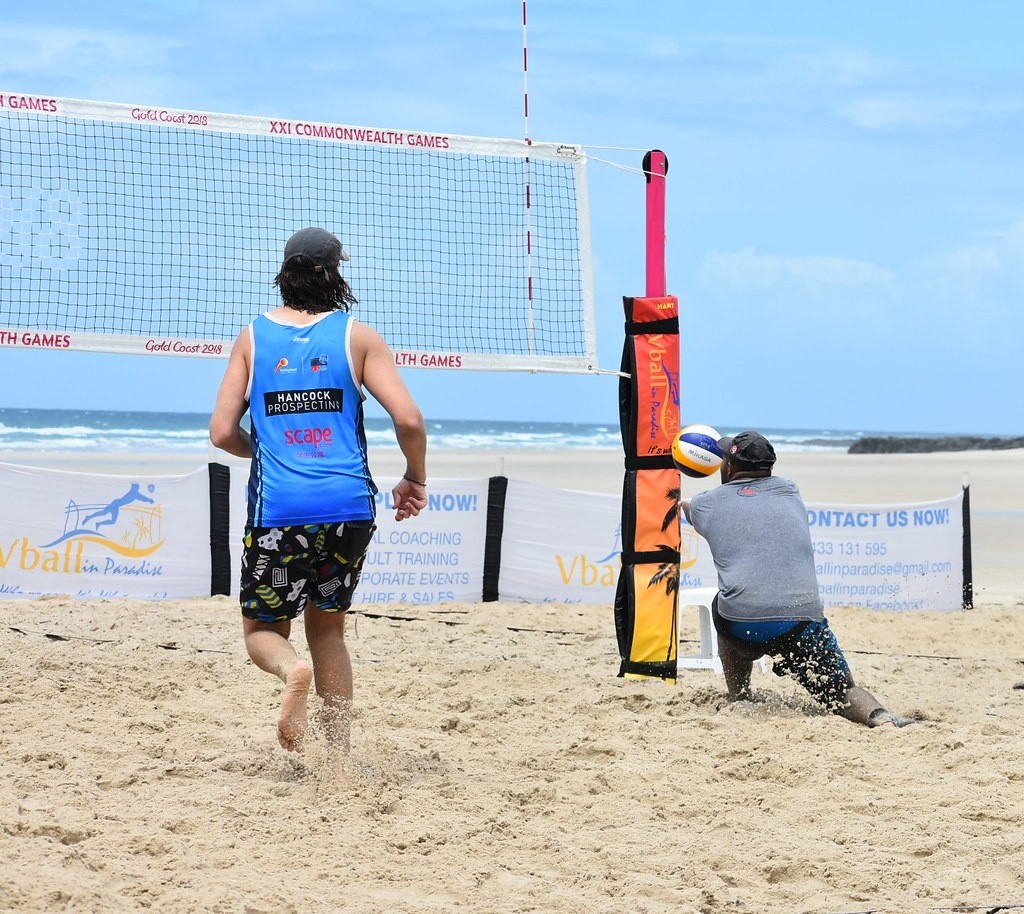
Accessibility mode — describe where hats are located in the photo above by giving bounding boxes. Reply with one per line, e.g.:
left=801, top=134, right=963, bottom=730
left=716, top=431, right=776, bottom=470
left=284, top=227, right=349, bottom=273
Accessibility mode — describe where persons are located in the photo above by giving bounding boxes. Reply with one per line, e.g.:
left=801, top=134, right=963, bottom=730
left=209, top=225, right=430, bottom=764
left=680, top=433, right=920, bottom=727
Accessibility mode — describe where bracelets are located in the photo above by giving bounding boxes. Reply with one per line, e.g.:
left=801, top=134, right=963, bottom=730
left=403, top=474, right=428, bottom=487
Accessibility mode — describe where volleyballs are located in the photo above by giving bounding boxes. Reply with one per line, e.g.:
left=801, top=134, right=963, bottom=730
left=671, top=425, right=724, bottom=478
